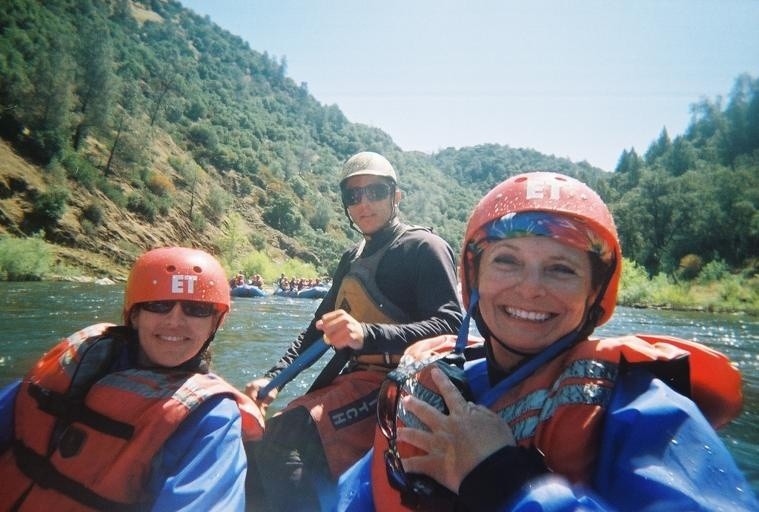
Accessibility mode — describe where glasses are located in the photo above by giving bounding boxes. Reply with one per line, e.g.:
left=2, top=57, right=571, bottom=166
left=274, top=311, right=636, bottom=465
left=138, top=301, right=213, bottom=317
left=342, top=184, right=389, bottom=206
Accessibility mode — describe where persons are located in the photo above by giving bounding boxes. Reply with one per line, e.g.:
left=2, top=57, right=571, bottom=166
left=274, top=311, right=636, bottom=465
left=0, top=247, right=248, bottom=512
left=230, top=270, right=263, bottom=289
left=245, top=151, right=464, bottom=512
left=277, top=273, right=331, bottom=291
left=371, top=171, right=759, bottom=512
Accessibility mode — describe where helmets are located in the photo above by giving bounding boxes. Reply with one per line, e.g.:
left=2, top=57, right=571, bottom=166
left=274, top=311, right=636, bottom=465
left=341, top=151, right=396, bottom=185
left=461, top=173, right=621, bottom=326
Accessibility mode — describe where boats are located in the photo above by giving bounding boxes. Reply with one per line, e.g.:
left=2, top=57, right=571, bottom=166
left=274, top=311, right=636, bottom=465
left=229, top=283, right=269, bottom=298
left=273, top=283, right=331, bottom=300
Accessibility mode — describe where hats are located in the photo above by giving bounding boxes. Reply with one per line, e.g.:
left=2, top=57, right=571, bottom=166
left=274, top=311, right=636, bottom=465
left=124, top=248, right=230, bottom=327
